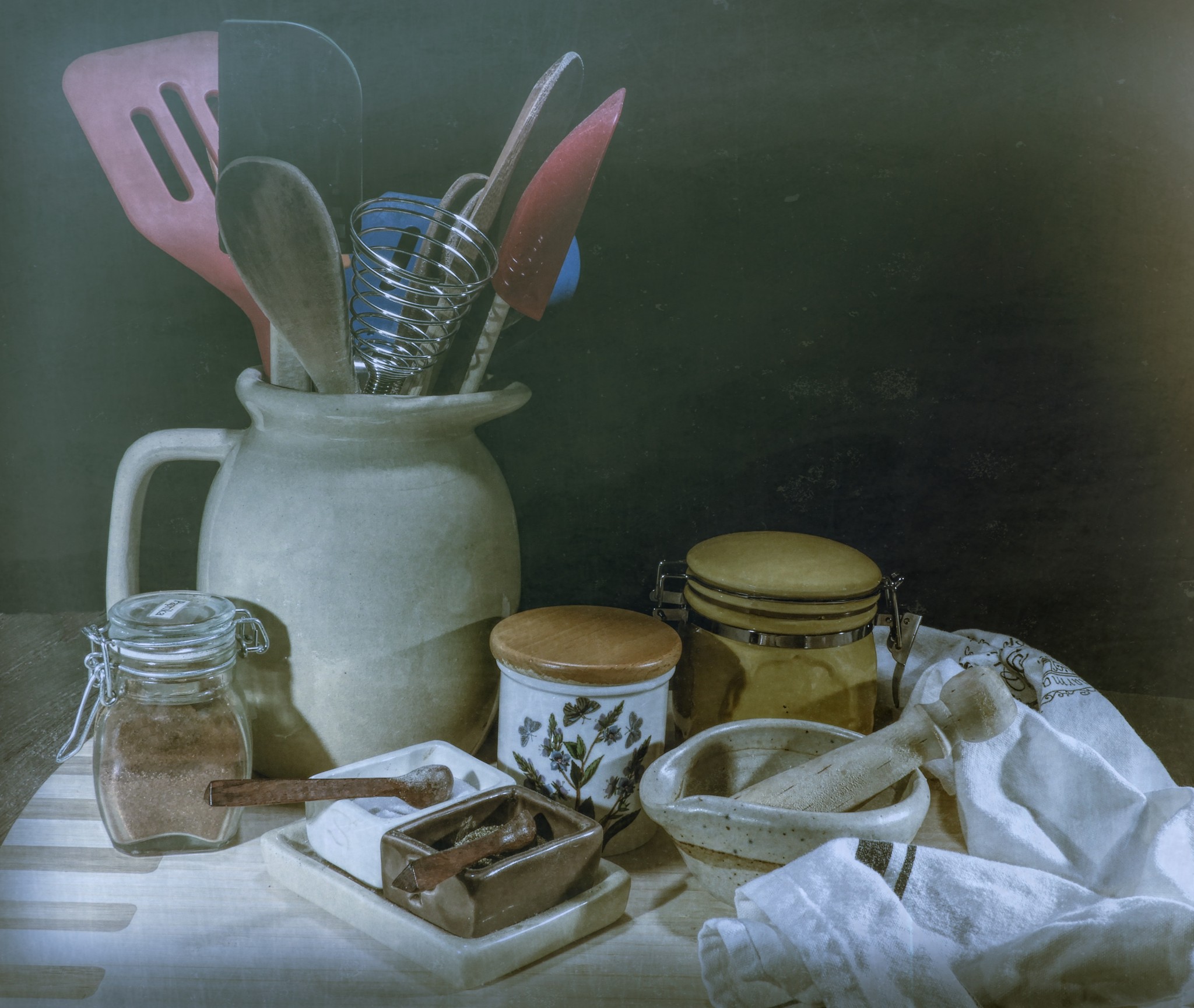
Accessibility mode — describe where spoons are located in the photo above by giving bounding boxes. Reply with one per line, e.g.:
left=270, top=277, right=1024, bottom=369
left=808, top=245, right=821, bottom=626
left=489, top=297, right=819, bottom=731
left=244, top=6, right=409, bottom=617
left=204, top=762, right=455, bottom=811
left=391, top=809, right=536, bottom=894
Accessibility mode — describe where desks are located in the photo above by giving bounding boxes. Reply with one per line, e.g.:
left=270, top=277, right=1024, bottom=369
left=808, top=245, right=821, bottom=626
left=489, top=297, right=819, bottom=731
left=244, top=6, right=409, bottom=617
left=1, top=612, right=1194, bottom=1007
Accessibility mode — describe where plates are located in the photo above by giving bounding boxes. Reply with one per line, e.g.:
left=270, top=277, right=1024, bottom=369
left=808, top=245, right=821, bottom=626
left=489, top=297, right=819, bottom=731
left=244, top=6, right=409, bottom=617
left=262, top=816, right=632, bottom=992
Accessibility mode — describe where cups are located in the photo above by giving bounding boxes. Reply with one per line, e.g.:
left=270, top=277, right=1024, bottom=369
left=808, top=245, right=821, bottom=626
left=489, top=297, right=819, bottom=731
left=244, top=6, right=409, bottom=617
left=495, top=653, right=679, bottom=853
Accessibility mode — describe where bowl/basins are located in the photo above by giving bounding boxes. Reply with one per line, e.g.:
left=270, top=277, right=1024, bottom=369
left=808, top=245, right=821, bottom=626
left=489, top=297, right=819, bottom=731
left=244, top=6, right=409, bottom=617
left=638, top=716, right=929, bottom=907
left=306, top=739, right=518, bottom=887
left=380, top=786, right=604, bottom=938
